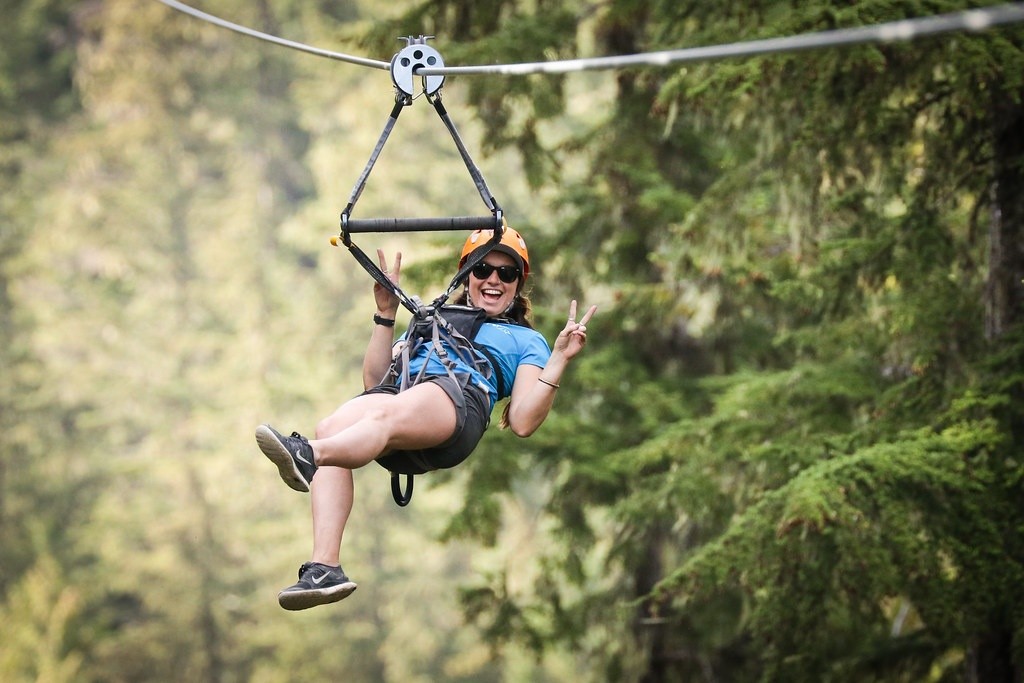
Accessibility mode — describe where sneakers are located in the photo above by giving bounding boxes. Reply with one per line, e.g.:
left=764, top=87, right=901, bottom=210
left=278, top=561, right=358, bottom=611
left=254, top=423, right=317, bottom=493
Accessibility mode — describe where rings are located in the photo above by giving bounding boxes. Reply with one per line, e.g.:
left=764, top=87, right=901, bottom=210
left=568, top=318, right=575, bottom=321
left=579, top=321, right=585, bottom=326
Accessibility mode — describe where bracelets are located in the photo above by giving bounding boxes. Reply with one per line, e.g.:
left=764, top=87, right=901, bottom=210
left=538, top=377, right=560, bottom=388
left=374, top=313, right=395, bottom=327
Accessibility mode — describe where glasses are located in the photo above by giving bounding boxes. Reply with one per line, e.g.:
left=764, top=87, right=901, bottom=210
left=472, top=260, right=521, bottom=283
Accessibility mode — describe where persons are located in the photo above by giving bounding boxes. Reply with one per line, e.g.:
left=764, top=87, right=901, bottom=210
left=254, top=226, right=598, bottom=612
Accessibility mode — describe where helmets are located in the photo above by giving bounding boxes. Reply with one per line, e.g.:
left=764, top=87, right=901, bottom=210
left=458, top=225, right=530, bottom=282
